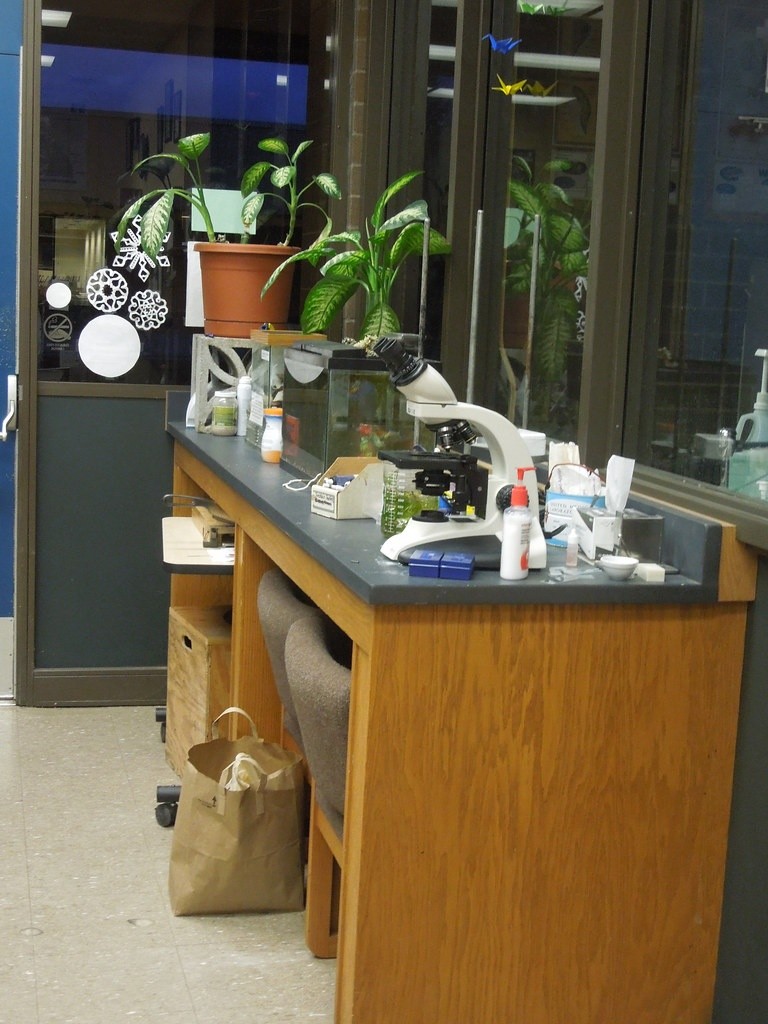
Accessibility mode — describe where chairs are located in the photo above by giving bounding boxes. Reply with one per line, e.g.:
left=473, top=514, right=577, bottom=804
left=256, top=568, right=330, bottom=787
left=283, top=619, right=351, bottom=958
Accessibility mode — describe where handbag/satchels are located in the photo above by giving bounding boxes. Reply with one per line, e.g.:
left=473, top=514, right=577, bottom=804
left=168, top=707, right=308, bottom=916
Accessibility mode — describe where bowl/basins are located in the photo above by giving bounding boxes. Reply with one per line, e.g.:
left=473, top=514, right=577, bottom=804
left=600, top=556, right=639, bottom=581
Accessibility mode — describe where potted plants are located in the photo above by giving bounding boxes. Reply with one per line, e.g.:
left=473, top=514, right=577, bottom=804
left=261, top=168, right=452, bottom=353
left=116, top=132, right=343, bottom=340
left=506, top=158, right=587, bottom=429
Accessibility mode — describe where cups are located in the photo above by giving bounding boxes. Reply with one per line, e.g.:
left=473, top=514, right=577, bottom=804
left=380, top=461, right=438, bottom=539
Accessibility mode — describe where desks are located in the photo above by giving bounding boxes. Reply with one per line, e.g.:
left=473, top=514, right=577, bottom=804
left=166, top=422, right=759, bottom=1024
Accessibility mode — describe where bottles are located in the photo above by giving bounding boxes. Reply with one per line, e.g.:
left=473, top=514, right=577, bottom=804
left=211, top=390, right=238, bottom=436
left=260, top=408, right=283, bottom=464
left=566, top=528, right=578, bottom=566
left=236, top=376, right=251, bottom=436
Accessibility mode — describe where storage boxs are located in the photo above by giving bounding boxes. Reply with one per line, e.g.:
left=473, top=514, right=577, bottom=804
left=281, top=350, right=420, bottom=482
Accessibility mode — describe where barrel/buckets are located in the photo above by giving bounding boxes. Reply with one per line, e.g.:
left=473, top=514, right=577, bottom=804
left=544, top=462, right=604, bottom=547
